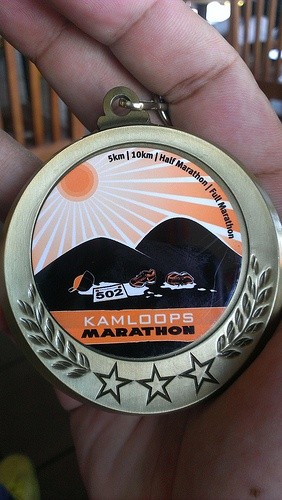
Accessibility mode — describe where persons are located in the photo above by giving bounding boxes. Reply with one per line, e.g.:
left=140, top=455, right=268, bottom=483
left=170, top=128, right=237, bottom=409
left=0, top=0, right=282, bottom=500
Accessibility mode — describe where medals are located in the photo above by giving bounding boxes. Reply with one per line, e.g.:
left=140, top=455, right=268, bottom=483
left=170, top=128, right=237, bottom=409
left=1, top=86, right=282, bottom=416
